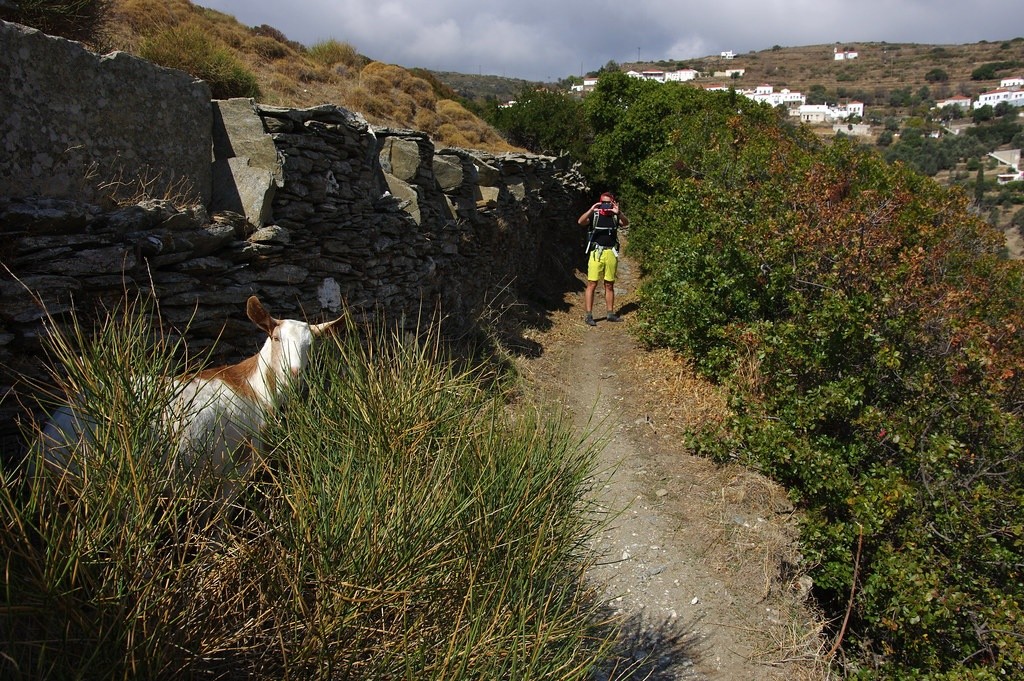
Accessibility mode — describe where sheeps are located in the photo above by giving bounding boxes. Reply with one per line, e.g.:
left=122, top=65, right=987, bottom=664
left=31, top=294, right=346, bottom=525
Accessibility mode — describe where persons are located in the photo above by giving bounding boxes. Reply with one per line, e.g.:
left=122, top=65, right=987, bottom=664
left=578, top=192, right=630, bottom=326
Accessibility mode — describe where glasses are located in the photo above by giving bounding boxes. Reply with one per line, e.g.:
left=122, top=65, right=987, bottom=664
left=602, top=201, right=611, bottom=204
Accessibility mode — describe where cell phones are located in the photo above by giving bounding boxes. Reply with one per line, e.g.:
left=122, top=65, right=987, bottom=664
left=601, top=204, right=613, bottom=209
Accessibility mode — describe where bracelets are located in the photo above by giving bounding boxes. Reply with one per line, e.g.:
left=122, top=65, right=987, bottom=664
left=616, top=211, right=621, bottom=215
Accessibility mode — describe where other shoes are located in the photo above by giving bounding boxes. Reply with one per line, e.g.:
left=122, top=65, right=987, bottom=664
left=585, top=314, right=596, bottom=326
left=607, top=314, right=623, bottom=322
